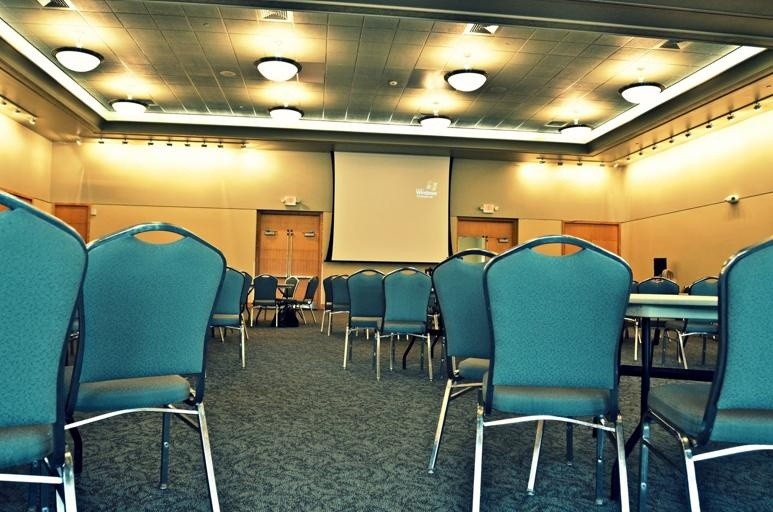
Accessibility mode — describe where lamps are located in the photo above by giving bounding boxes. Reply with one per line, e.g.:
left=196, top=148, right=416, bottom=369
left=51, top=47, right=104, bottom=73
left=618, top=81, right=665, bottom=104
left=253, top=56, right=302, bottom=82
left=108, top=99, right=149, bottom=118
left=418, top=115, right=451, bottom=130
left=558, top=124, right=593, bottom=140
left=443, top=69, right=488, bottom=92
left=268, top=106, right=304, bottom=120
